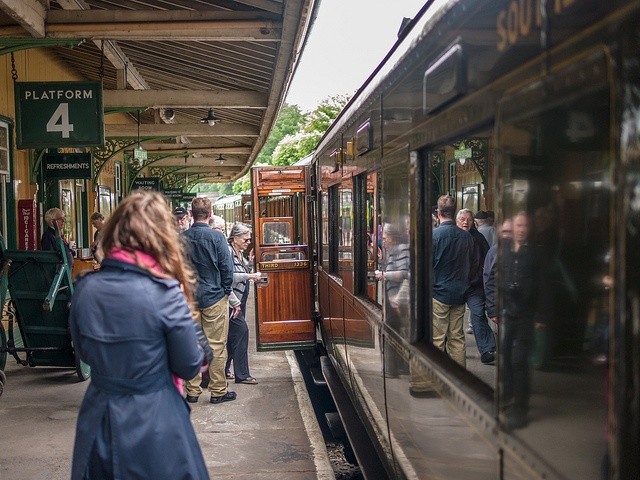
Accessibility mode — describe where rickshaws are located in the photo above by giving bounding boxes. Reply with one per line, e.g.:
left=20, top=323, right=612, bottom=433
left=0, top=219, right=92, bottom=380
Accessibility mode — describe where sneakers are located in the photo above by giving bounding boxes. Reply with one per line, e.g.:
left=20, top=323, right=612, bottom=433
left=212, top=391, right=236, bottom=403
left=186, top=394, right=197, bottom=403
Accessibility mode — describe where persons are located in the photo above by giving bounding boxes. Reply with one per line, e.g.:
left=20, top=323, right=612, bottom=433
left=179, top=197, right=237, bottom=403
left=431, top=194, right=480, bottom=369
left=374, top=222, right=409, bottom=378
left=90, top=211, right=103, bottom=254
left=456, top=207, right=497, bottom=364
left=43, top=208, right=73, bottom=273
left=465, top=211, right=496, bottom=335
left=500, top=211, right=543, bottom=431
left=482, top=218, right=514, bottom=340
left=173, top=208, right=190, bottom=234
left=339, top=209, right=352, bottom=244
left=220, top=224, right=270, bottom=384
left=66, top=188, right=211, bottom=479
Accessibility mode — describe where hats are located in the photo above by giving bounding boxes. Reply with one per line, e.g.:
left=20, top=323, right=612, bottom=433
left=474, top=210, right=488, bottom=219
left=173, top=208, right=187, bottom=218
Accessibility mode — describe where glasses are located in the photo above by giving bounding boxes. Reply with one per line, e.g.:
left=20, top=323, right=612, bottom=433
left=243, top=238, right=251, bottom=243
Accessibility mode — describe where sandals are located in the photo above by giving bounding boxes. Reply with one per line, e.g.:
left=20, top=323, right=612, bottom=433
left=242, top=376, right=258, bottom=384
left=225, top=372, right=234, bottom=378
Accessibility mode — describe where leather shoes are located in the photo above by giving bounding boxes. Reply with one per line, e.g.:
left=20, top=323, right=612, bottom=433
left=480, top=351, right=495, bottom=363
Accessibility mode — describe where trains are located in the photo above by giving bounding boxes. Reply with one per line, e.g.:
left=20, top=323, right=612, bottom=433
left=211, top=0, right=638, bottom=479
